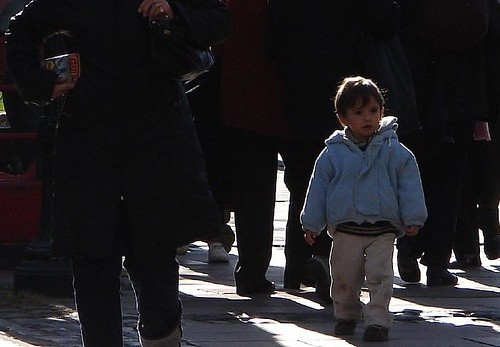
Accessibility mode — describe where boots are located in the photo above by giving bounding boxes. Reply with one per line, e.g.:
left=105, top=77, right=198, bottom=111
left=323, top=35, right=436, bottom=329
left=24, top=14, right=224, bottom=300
left=138, top=298, right=183, bottom=347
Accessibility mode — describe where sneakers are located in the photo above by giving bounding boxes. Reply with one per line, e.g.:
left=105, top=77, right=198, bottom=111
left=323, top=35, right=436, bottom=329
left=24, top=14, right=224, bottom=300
left=306, top=258, right=332, bottom=293
left=462, top=228, right=500, bottom=266
left=397, top=253, right=458, bottom=286
left=335, top=319, right=388, bottom=342
left=177, top=241, right=231, bottom=263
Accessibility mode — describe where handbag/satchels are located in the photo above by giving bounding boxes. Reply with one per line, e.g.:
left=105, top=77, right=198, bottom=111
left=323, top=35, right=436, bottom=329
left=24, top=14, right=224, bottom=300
left=149, top=16, right=216, bottom=85
left=360, top=29, right=420, bottom=136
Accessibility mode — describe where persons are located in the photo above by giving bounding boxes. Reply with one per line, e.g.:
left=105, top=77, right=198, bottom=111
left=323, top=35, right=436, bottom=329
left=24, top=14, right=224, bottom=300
left=171, top=0, right=500, bottom=297
left=4, top=0, right=226, bottom=347
left=299, top=75, right=426, bottom=342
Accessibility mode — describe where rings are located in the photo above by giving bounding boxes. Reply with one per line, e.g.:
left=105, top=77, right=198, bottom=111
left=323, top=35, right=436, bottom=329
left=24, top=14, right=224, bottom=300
left=151, top=0, right=169, bottom=21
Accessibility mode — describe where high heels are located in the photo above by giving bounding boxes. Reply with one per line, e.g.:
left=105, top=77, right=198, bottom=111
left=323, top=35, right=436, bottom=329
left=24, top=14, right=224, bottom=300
left=235, top=264, right=321, bottom=295
